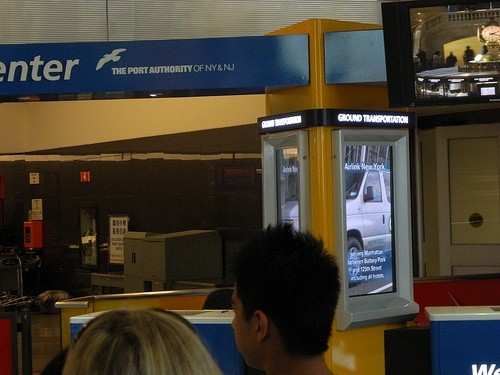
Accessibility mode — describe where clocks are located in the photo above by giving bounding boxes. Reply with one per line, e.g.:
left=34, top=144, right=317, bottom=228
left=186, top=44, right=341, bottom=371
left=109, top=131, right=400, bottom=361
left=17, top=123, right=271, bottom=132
left=481, top=25, right=500, bottom=43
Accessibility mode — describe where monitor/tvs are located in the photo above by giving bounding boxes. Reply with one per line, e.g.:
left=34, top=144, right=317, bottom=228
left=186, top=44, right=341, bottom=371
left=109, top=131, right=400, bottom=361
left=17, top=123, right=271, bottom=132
left=342, top=140, right=404, bottom=298
left=270, top=142, right=302, bottom=229
left=381, top=0, right=500, bottom=107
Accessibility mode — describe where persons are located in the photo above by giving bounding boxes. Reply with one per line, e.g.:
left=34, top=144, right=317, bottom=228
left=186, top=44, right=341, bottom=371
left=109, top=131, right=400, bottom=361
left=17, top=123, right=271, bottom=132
left=413, top=45, right=487, bottom=71
left=62, top=311, right=221, bottom=373
left=232, top=222, right=343, bottom=375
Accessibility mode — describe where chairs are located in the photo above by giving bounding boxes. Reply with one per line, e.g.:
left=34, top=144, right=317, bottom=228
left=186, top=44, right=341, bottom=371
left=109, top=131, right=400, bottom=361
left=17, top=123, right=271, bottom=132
left=202, top=289, right=236, bottom=310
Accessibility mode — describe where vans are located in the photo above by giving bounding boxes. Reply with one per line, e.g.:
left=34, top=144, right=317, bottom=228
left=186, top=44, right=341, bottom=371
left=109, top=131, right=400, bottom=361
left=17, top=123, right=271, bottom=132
left=279, top=163, right=393, bottom=287
